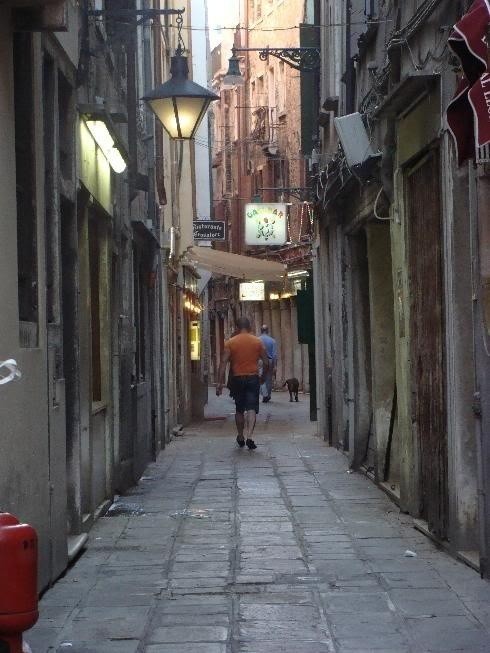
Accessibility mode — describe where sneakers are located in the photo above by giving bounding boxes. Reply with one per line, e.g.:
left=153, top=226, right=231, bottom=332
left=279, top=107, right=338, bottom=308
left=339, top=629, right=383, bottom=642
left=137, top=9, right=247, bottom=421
left=236, top=434, right=257, bottom=450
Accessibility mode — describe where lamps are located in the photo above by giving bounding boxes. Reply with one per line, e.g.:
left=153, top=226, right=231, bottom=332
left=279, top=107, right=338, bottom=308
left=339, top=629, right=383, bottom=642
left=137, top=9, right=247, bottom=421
left=77, top=101, right=130, bottom=175
left=224, top=43, right=321, bottom=96
left=82, top=4, right=225, bottom=141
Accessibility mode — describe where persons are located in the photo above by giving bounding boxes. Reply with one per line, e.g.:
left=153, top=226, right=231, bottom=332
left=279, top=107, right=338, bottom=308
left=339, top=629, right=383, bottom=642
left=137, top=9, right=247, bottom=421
left=258, top=324, right=280, bottom=403
left=215, top=317, right=269, bottom=449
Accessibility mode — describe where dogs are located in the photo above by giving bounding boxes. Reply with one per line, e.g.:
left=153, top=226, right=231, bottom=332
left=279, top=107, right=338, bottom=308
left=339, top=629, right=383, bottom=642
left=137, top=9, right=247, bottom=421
left=282, top=378, right=299, bottom=402
